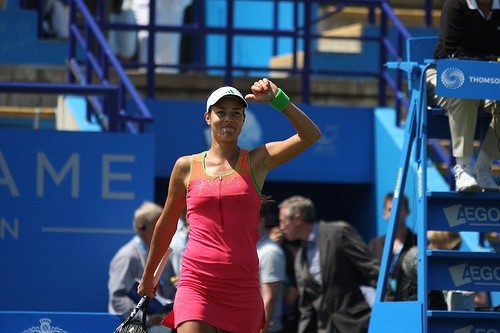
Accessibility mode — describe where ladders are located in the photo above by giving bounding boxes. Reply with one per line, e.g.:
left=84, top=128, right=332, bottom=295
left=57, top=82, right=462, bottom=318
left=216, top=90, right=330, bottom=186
left=367, top=60, right=499, bottom=333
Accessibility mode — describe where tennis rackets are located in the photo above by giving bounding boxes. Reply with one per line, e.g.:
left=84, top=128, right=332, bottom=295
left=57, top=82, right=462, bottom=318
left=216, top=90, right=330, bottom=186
left=113, top=248, right=173, bottom=333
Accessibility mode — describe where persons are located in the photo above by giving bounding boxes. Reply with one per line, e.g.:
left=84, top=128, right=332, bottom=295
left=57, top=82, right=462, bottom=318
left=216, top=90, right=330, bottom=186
left=137, top=78, right=321, bottom=333
left=270, top=196, right=397, bottom=333
left=256, top=207, right=285, bottom=332
left=168, top=208, right=193, bottom=281
left=426, top=0, right=500, bottom=193
left=108, top=200, right=181, bottom=333
left=368, top=192, right=500, bottom=312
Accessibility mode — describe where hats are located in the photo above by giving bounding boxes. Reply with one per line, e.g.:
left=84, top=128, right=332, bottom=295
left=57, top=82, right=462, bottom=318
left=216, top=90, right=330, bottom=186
left=207, top=86, right=249, bottom=112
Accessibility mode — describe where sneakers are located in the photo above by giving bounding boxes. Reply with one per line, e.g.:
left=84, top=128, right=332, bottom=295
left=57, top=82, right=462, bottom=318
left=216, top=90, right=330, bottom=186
left=473, top=163, right=499, bottom=191
left=454, top=163, right=482, bottom=192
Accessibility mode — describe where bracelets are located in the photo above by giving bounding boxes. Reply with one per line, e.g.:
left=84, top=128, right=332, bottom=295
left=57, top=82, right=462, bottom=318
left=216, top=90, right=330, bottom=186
left=268, top=88, right=290, bottom=112
